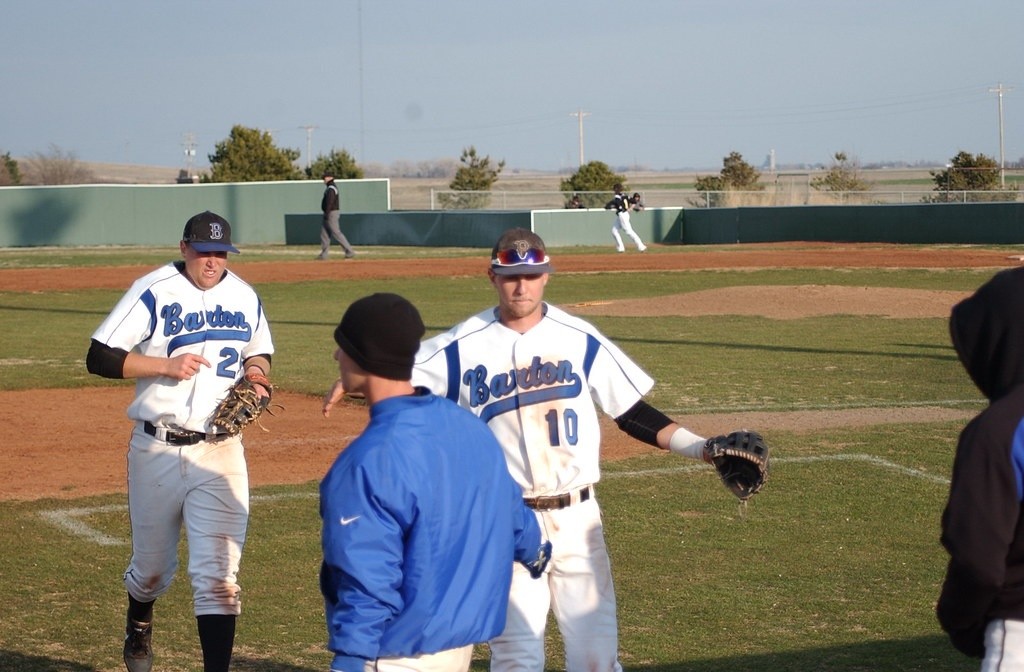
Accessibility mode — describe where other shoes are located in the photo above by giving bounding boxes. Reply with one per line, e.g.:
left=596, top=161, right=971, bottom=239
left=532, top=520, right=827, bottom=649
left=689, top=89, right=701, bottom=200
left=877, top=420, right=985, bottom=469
left=345, top=250, right=354, bottom=259
left=317, top=251, right=329, bottom=260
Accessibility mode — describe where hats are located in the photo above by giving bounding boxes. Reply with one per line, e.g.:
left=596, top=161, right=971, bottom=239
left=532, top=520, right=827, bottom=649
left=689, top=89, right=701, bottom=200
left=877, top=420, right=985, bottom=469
left=321, top=171, right=334, bottom=179
left=334, top=293, right=425, bottom=381
left=184, top=210, right=240, bottom=253
left=491, top=229, right=552, bottom=275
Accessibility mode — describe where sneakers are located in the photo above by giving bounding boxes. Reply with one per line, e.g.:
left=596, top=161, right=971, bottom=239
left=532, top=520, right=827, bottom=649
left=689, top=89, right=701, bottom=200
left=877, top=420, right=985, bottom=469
left=124, top=615, right=153, bottom=672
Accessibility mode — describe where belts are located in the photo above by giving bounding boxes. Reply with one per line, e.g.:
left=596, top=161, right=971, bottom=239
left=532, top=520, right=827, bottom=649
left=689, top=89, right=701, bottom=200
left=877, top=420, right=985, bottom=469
left=522, top=488, right=589, bottom=510
left=144, top=421, right=227, bottom=445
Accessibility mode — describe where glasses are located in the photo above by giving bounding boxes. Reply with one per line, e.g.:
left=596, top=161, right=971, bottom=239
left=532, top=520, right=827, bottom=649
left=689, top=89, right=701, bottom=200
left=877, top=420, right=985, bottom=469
left=491, top=248, right=550, bottom=266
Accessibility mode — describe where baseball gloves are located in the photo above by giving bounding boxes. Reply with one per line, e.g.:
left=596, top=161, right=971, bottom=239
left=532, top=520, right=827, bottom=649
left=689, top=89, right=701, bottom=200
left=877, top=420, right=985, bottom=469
left=703, top=428, right=771, bottom=518
left=207, top=373, right=286, bottom=438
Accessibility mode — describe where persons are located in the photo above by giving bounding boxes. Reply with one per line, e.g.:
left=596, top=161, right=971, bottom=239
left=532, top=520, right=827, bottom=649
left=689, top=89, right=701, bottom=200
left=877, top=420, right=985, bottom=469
left=605, top=184, right=647, bottom=253
left=318, top=294, right=553, bottom=672
left=566, top=196, right=583, bottom=208
left=631, top=193, right=644, bottom=211
left=316, top=172, right=355, bottom=259
left=935, top=266, right=1024, bottom=672
left=86, top=211, right=274, bottom=672
left=323, top=228, right=768, bottom=672
